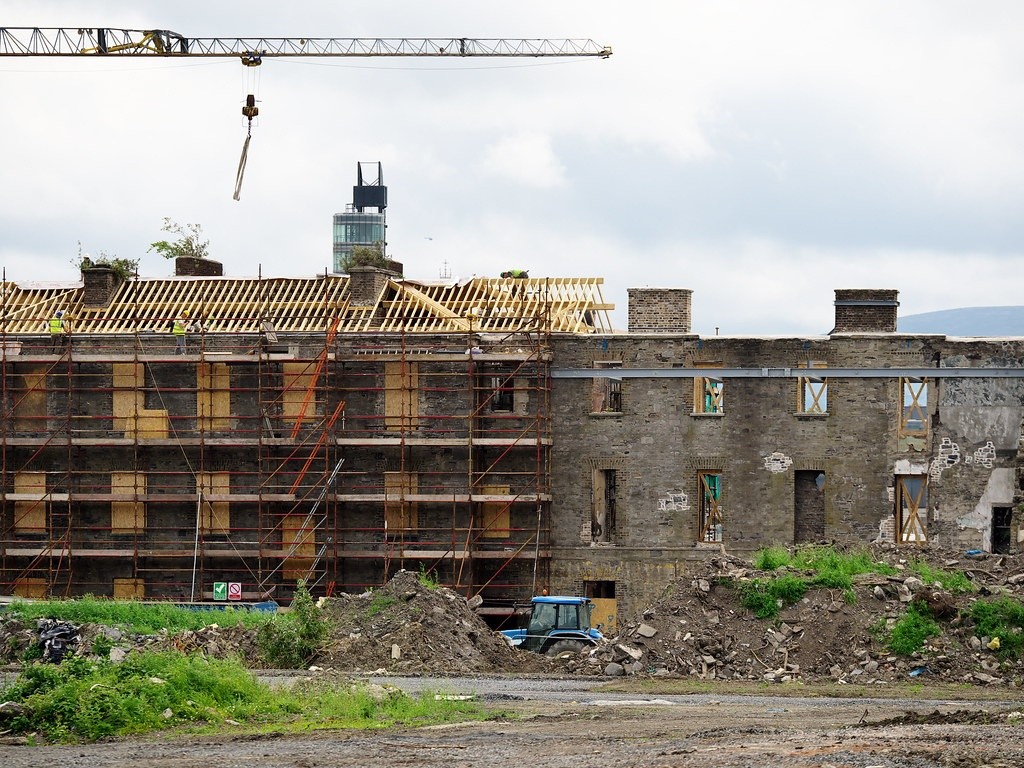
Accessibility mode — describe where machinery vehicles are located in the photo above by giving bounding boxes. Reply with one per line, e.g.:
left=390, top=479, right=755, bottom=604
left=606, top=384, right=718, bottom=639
left=497, top=596, right=604, bottom=659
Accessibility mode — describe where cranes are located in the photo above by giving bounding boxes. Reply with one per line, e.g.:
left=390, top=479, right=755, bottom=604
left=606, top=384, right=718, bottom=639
left=0, top=24, right=612, bottom=118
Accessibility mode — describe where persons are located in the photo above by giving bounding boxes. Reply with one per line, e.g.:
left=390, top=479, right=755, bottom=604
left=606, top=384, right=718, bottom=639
left=170, top=309, right=215, bottom=355
left=500, top=269, right=529, bottom=301
left=49, top=311, right=68, bottom=355
left=579, top=310, right=597, bottom=327
